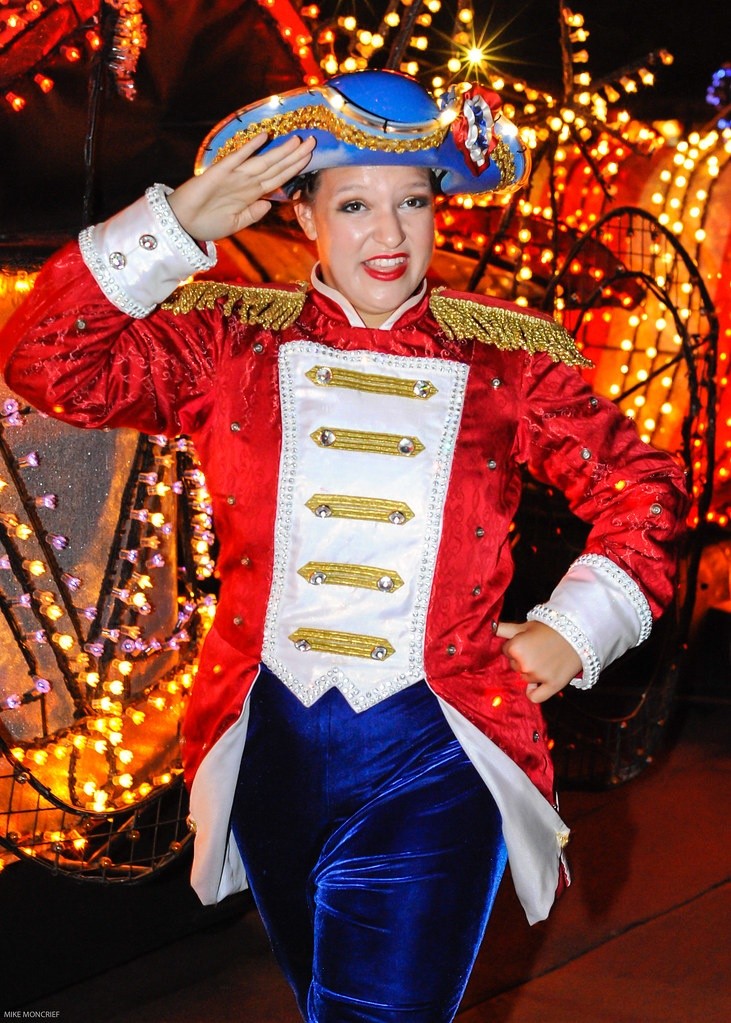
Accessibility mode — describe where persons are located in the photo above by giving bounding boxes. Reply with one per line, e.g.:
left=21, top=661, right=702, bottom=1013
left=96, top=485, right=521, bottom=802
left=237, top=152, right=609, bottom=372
left=0, top=71, right=689, bottom=1023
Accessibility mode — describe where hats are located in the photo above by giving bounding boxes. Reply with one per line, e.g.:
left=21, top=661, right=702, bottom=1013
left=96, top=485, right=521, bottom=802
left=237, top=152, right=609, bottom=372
left=194, top=67, right=526, bottom=203
